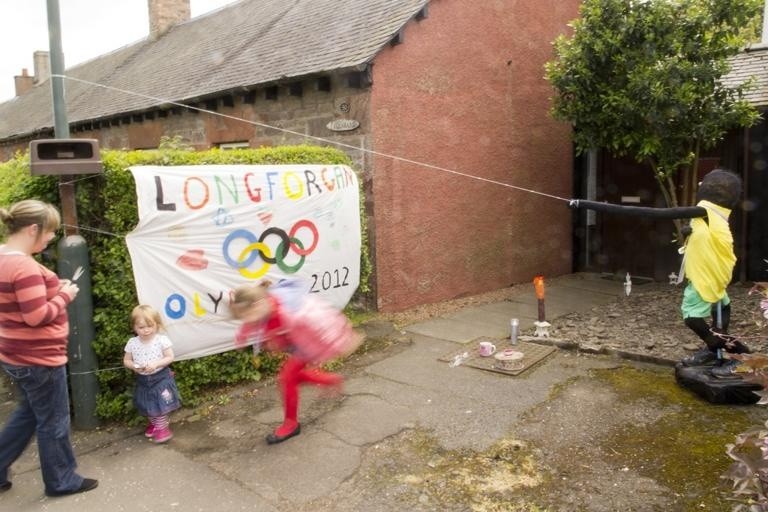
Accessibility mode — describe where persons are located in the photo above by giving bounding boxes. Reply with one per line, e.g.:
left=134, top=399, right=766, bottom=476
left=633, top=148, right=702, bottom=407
left=122, top=303, right=184, bottom=443
left=569, top=168, right=757, bottom=370
left=0, top=199, right=99, bottom=497
left=228, top=279, right=366, bottom=444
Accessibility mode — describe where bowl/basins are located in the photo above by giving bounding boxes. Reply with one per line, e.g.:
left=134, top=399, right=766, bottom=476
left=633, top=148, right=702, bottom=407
left=494, top=351, right=524, bottom=370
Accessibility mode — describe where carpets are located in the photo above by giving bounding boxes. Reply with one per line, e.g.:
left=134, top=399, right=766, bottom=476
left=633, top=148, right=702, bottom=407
left=436, top=336, right=557, bottom=376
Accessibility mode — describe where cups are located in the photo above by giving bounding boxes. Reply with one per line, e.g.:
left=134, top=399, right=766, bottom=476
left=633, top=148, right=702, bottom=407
left=479, top=342, right=495, bottom=357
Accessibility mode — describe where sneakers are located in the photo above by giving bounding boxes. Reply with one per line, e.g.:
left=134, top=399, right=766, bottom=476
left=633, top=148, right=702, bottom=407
left=712, top=356, right=749, bottom=379
left=681, top=347, right=715, bottom=366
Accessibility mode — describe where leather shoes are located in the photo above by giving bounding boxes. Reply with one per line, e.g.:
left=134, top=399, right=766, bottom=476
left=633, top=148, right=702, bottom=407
left=266, top=422, right=300, bottom=444
left=145, top=424, right=155, bottom=437
left=45, top=477, right=98, bottom=496
left=155, top=427, right=173, bottom=444
left=0, top=480, right=10, bottom=491
